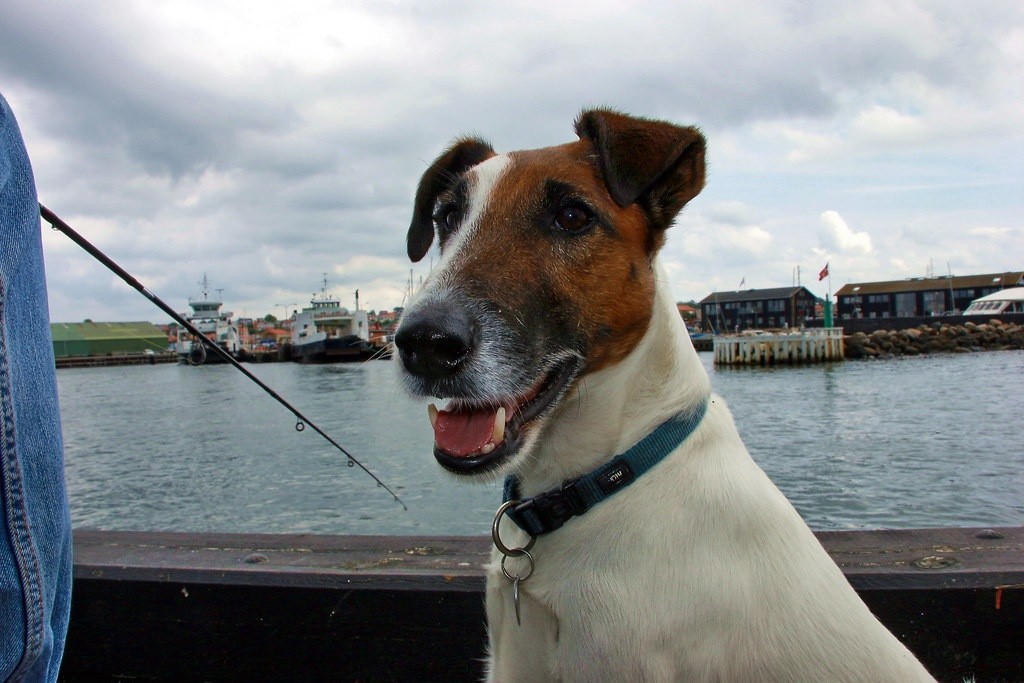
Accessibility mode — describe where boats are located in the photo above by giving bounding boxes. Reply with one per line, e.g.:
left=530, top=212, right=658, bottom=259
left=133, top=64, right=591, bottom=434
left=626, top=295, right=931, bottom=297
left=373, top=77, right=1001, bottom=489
left=962, top=287, right=1024, bottom=314
left=176, top=270, right=240, bottom=363
left=290, top=272, right=381, bottom=362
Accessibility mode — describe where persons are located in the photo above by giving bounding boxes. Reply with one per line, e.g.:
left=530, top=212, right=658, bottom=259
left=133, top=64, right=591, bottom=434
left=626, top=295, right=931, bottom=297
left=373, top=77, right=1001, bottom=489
left=0, top=93, right=72, bottom=683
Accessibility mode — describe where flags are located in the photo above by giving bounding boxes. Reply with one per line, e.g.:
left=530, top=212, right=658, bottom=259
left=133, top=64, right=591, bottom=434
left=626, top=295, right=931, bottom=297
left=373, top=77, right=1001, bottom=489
left=819, top=267, right=828, bottom=281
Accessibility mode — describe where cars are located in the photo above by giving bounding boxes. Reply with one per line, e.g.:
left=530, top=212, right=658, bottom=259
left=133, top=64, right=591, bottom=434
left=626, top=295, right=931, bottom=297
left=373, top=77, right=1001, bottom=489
left=260, top=340, right=277, bottom=348
left=143, top=349, right=154, bottom=356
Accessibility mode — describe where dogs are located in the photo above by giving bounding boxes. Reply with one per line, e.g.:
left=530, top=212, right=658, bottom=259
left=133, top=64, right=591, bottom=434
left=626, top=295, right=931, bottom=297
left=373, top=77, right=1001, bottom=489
left=391, top=106, right=935, bottom=682
